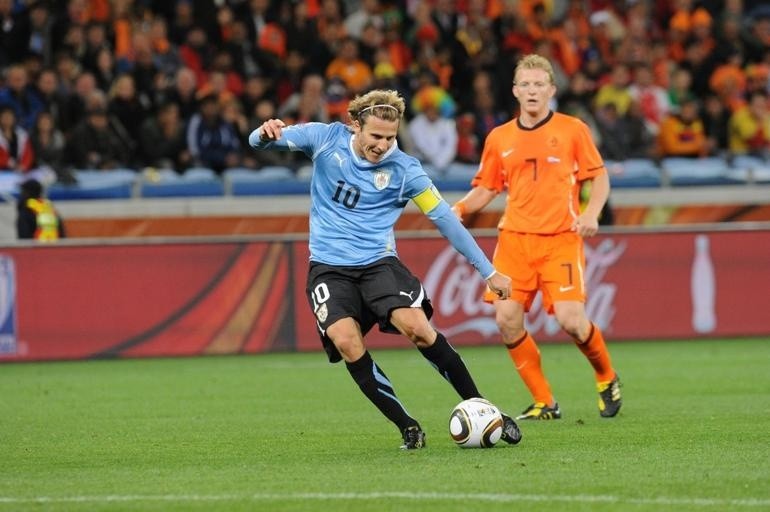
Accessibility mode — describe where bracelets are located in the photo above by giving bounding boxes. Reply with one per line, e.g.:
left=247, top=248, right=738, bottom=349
left=454, top=202, right=466, bottom=216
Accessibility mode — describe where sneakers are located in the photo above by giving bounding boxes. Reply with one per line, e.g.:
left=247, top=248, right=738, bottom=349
left=500, top=413, right=521, bottom=444
left=597, top=372, right=620, bottom=418
left=400, top=428, right=424, bottom=449
left=519, top=403, right=561, bottom=420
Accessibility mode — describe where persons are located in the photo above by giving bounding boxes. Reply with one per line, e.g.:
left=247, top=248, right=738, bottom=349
left=249, top=89, right=522, bottom=452
left=15, top=179, right=66, bottom=241
left=1, top=1, right=770, bottom=175
left=578, top=178, right=614, bottom=226
left=447, top=53, right=623, bottom=421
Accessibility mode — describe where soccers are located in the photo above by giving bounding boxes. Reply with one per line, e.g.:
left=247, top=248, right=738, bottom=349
left=449, top=397, right=503, bottom=448
